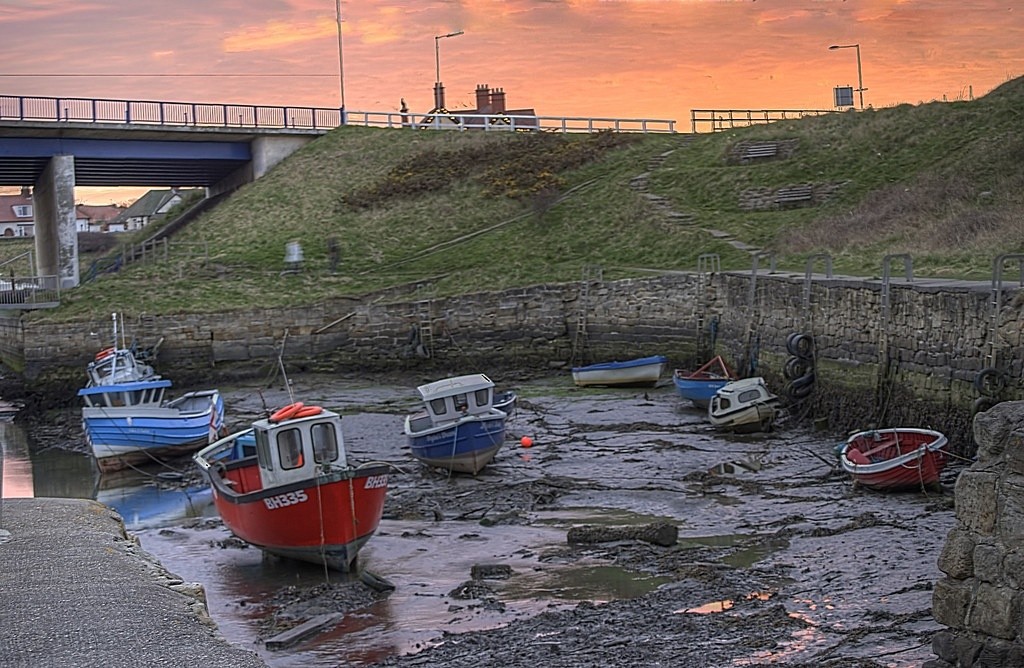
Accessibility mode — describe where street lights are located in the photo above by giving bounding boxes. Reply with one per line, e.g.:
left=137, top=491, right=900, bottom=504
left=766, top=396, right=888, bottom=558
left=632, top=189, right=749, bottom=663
left=435, top=30, right=464, bottom=114
left=829, top=43, right=863, bottom=113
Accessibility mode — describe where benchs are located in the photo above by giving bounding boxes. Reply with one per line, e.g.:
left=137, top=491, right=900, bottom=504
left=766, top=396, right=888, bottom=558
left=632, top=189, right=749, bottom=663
left=773, top=185, right=815, bottom=210
left=740, top=142, right=779, bottom=165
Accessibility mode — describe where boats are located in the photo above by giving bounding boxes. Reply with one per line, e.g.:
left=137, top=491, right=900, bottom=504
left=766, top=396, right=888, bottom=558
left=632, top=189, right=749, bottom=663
left=673, top=354, right=733, bottom=407
left=707, top=376, right=781, bottom=435
left=403, top=372, right=517, bottom=477
left=192, top=406, right=390, bottom=570
left=77, top=311, right=225, bottom=476
left=839, top=429, right=949, bottom=491
left=572, top=354, right=669, bottom=388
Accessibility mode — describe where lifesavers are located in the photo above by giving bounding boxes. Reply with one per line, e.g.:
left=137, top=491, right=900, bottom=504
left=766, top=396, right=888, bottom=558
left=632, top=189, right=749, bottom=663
left=970, top=397, right=997, bottom=419
left=416, top=343, right=431, bottom=360
left=973, top=368, right=1006, bottom=396
left=96, top=347, right=114, bottom=361
left=782, top=333, right=816, bottom=404
left=268, top=401, right=323, bottom=423
